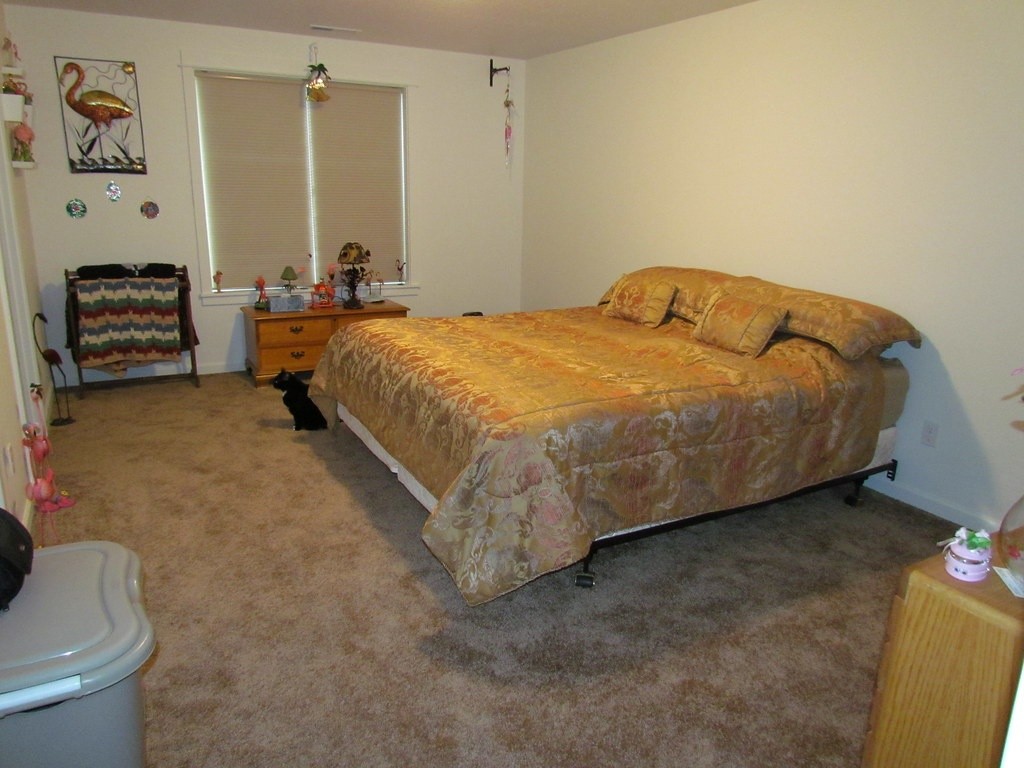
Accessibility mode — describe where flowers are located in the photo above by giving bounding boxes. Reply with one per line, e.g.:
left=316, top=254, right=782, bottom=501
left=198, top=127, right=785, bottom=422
left=2, top=78, right=36, bottom=162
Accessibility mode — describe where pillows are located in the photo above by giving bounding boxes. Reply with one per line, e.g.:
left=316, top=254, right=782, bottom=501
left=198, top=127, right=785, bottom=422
left=726, top=275, right=921, bottom=360
left=598, top=266, right=738, bottom=325
left=692, top=286, right=787, bottom=359
left=602, top=273, right=676, bottom=327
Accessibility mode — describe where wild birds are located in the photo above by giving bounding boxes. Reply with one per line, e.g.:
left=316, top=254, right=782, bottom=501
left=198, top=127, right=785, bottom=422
left=58, top=61, right=137, bottom=169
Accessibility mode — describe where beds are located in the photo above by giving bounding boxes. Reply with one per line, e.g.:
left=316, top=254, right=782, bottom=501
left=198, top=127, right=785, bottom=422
left=305, top=302, right=910, bottom=606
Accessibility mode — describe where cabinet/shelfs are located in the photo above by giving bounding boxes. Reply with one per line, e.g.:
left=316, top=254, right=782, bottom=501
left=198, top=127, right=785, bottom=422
left=64, top=263, right=199, bottom=399
left=240, top=298, right=411, bottom=388
left=0, top=66, right=39, bottom=169
left=859, top=526, right=1024, bottom=766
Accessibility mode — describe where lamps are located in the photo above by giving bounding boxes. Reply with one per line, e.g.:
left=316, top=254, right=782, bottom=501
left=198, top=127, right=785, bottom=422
left=337, top=242, right=370, bottom=309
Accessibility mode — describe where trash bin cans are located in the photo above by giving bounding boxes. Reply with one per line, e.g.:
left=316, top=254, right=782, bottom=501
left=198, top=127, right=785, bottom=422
left=0, top=541, right=159, bottom=768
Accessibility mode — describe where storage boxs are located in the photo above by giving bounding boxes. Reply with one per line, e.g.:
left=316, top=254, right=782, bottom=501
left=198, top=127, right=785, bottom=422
left=0, top=540, right=157, bottom=768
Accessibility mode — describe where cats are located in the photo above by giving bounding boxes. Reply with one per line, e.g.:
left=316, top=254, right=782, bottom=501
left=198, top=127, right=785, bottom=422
left=272, top=365, right=344, bottom=432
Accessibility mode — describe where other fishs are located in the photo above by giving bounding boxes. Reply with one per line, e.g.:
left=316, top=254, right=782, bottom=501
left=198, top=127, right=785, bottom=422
left=336, top=242, right=372, bottom=265
